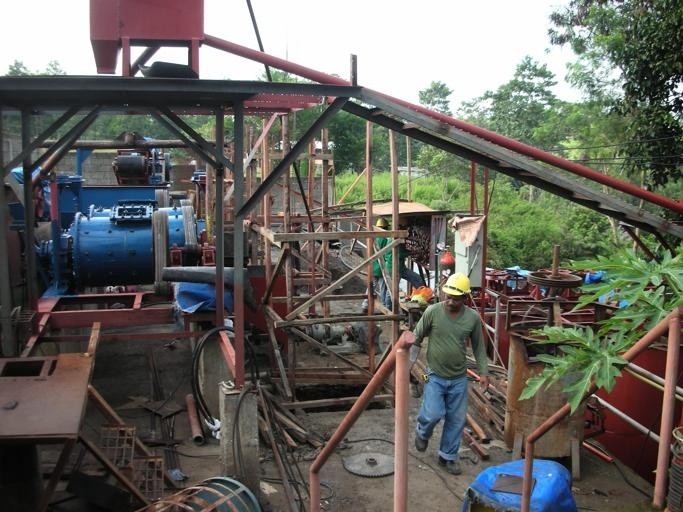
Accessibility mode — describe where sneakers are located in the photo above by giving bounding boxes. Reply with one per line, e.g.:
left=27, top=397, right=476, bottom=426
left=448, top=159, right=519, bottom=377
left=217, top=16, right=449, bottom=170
left=414, top=433, right=427, bottom=452
left=437, top=456, right=460, bottom=475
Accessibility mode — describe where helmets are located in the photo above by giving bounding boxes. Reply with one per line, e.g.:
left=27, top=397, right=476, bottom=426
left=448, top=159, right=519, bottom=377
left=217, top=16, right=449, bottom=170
left=441, top=274, right=470, bottom=295
left=375, top=219, right=390, bottom=227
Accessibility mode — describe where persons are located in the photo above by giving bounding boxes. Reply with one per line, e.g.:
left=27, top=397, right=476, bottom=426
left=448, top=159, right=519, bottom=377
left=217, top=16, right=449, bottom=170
left=359, top=216, right=388, bottom=313
left=408, top=272, right=490, bottom=476
left=384, top=218, right=438, bottom=310
